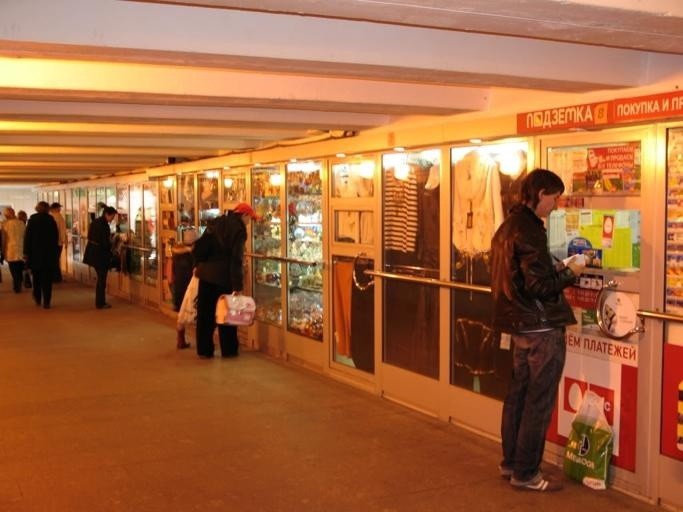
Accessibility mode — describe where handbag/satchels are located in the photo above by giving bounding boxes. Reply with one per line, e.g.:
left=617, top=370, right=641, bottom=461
left=22, top=271, right=31, bottom=289
left=213, top=290, right=257, bottom=328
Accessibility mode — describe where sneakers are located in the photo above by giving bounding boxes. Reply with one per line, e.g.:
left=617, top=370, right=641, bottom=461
left=34, top=299, right=53, bottom=309
left=498, top=458, right=514, bottom=481
left=96, top=302, right=112, bottom=309
left=506, top=469, right=564, bottom=491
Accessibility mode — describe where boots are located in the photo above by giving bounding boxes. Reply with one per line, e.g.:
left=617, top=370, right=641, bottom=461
left=175, top=327, right=191, bottom=349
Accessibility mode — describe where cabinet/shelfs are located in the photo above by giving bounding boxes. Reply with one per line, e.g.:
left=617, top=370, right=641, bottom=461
left=250, top=192, right=322, bottom=340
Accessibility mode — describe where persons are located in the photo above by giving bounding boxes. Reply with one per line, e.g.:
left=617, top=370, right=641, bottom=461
left=0, top=207, right=26, bottom=293
left=23, top=201, right=61, bottom=309
left=0, top=212, right=4, bottom=264
left=193, top=203, right=262, bottom=357
left=489, top=168, right=588, bottom=490
left=48, top=202, right=66, bottom=283
left=83, top=207, right=117, bottom=309
left=16, top=210, right=32, bottom=282
left=171, top=229, right=203, bottom=348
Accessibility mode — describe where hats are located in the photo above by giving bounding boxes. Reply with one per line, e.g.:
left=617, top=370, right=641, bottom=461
left=231, top=203, right=261, bottom=222
